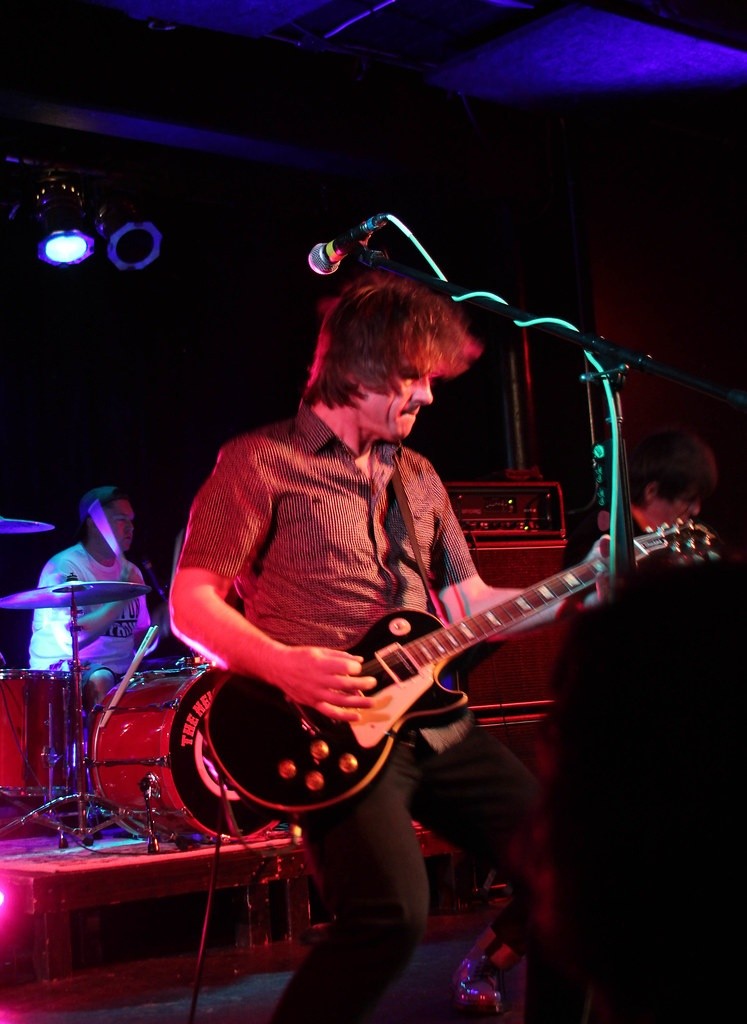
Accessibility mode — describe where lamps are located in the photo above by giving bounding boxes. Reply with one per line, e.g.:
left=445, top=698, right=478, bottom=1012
left=32, top=168, right=99, bottom=269
left=95, top=168, right=160, bottom=266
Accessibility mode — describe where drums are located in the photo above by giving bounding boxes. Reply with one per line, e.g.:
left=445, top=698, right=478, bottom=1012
left=0, top=668, right=78, bottom=798
left=85, top=666, right=283, bottom=840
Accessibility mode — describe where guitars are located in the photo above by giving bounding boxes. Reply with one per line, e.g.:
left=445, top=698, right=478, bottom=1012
left=202, top=514, right=716, bottom=813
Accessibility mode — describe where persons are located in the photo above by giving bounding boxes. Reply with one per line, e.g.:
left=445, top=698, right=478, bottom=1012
left=553, top=423, right=747, bottom=634
left=27, top=486, right=156, bottom=677
left=166, top=271, right=644, bottom=1023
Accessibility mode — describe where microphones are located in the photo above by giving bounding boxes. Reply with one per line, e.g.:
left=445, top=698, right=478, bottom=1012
left=309, top=212, right=391, bottom=275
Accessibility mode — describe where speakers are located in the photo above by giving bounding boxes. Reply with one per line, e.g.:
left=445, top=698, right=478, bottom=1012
left=453, top=477, right=571, bottom=707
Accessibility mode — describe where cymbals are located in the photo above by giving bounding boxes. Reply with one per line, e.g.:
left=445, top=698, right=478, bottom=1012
left=0, top=581, right=153, bottom=610
left=0, top=516, right=54, bottom=536
